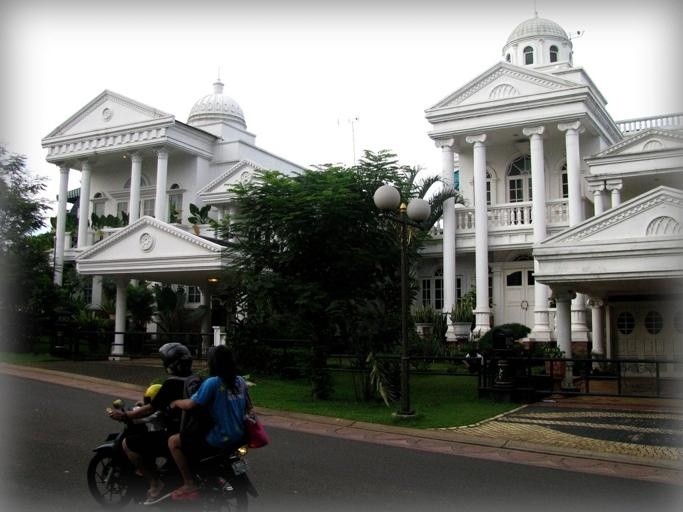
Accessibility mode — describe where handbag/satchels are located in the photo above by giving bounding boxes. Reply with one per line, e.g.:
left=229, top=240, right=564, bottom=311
left=239, top=377, right=269, bottom=448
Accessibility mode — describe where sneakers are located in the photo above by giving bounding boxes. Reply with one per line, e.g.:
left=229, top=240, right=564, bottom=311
left=171, top=486, right=201, bottom=502
left=143, top=485, right=175, bottom=505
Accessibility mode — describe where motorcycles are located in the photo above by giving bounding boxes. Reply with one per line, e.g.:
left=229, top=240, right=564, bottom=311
left=87, top=400, right=258, bottom=512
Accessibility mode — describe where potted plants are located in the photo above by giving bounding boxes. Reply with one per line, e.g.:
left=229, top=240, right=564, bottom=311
left=537, top=345, right=564, bottom=375
left=414, top=297, right=477, bottom=362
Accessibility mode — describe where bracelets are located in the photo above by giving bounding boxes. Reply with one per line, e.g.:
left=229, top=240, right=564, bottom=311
left=123, top=412, right=129, bottom=420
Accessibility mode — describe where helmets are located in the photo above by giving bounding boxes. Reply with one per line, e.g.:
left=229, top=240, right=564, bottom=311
left=157, top=342, right=193, bottom=376
left=143, top=383, right=162, bottom=404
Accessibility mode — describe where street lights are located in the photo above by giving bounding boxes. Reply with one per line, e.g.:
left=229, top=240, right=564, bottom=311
left=373, top=184, right=432, bottom=416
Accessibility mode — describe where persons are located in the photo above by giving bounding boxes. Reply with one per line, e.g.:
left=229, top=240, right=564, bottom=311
left=166, top=344, right=258, bottom=502
left=107, top=341, right=202, bottom=507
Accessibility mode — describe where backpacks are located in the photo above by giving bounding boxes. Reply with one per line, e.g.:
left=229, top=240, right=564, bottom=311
left=166, top=376, right=205, bottom=432
